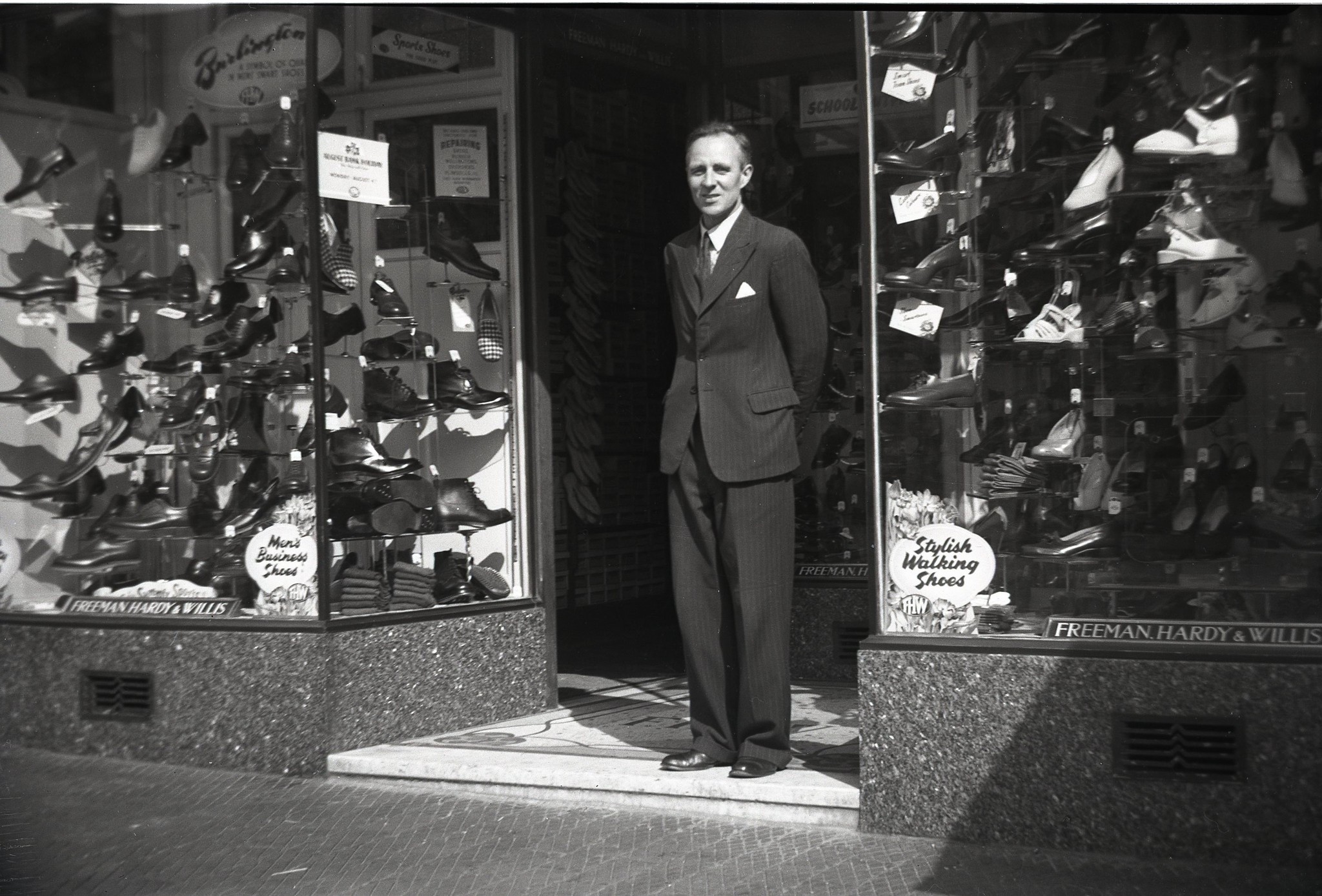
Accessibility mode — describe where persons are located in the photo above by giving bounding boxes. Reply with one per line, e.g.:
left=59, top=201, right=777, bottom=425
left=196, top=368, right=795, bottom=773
left=658, top=122, right=832, bottom=779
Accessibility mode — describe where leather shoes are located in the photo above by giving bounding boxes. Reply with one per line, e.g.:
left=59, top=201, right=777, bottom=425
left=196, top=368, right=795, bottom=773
left=876, top=10, right=1320, bottom=558
left=660, top=749, right=726, bottom=771
left=2, top=98, right=514, bottom=605
left=730, top=759, right=778, bottom=777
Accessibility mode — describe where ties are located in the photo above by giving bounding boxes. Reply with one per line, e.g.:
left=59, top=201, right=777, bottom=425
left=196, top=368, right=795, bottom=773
left=697, top=231, right=713, bottom=303
